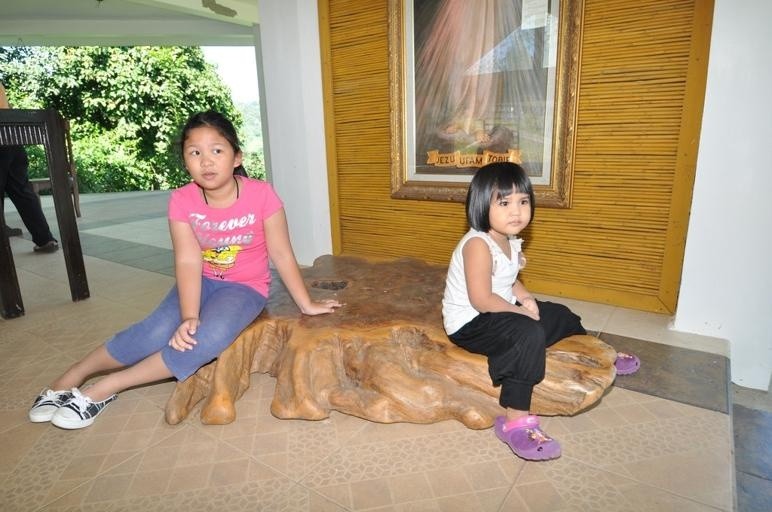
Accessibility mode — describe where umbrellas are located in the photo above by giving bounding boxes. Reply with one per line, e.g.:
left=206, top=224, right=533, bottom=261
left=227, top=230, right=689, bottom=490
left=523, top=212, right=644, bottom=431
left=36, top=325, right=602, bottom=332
left=6, top=225, right=22, bottom=236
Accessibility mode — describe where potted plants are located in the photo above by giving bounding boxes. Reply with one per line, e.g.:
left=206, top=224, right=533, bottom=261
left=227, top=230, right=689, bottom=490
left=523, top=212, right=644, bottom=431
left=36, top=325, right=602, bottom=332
left=384, top=2, right=585, bottom=211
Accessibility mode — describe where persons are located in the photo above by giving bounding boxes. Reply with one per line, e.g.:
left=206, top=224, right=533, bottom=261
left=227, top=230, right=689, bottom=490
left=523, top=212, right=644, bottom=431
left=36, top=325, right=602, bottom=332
left=441, top=162, right=640, bottom=461
left=29, top=111, right=343, bottom=430
left=0, top=81, right=58, bottom=253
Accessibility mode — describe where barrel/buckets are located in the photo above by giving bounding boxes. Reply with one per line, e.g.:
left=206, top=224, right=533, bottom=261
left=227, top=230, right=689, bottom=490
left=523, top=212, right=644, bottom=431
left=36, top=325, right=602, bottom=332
left=495, top=416, right=561, bottom=460
left=615, top=352, right=640, bottom=374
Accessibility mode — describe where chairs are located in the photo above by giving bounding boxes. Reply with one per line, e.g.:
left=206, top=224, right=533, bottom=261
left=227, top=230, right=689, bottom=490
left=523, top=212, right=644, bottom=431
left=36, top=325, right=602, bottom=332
left=29, top=387, right=72, bottom=423
left=51, top=383, right=118, bottom=429
left=34, top=241, right=58, bottom=252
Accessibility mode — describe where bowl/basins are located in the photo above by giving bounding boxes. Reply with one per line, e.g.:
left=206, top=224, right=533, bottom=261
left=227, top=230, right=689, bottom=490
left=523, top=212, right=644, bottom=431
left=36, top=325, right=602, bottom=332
left=203, top=177, right=240, bottom=206
